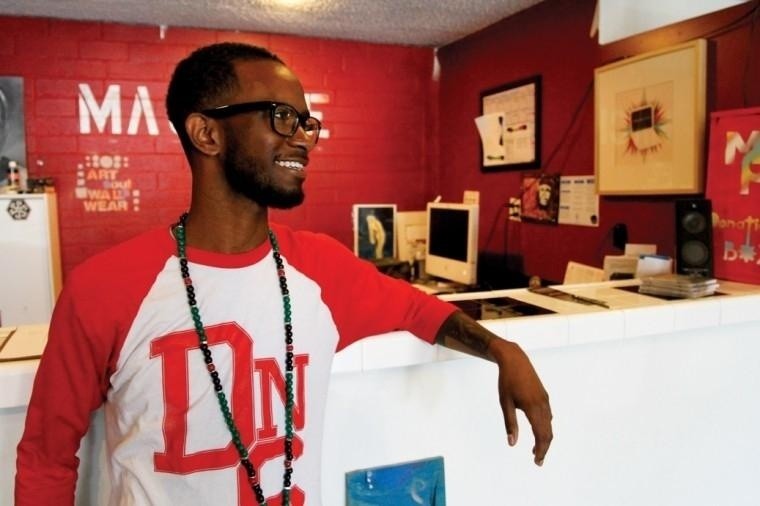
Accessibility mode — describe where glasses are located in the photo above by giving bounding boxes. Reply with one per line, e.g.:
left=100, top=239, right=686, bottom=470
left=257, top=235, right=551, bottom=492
left=201, top=100, right=322, bottom=144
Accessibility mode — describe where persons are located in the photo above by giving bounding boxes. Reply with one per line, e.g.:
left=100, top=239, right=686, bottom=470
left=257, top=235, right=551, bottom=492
left=14, top=43, right=553, bottom=506
left=522, top=178, right=557, bottom=222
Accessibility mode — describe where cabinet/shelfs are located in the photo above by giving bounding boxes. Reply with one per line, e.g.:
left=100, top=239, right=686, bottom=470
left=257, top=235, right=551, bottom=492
left=0, top=187, right=62, bottom=327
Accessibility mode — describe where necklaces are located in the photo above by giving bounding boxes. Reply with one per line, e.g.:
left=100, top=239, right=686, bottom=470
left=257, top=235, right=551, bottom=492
left=176, top=207, right=297, bottom=505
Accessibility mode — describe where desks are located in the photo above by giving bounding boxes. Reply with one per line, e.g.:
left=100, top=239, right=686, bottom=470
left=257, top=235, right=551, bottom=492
left=0, top=273, right=759, bottom=506
left=376, top=250, right=559, bottom=294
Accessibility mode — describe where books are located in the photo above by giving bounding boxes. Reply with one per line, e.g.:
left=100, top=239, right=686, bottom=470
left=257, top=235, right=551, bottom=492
left=637, top=272, right=721, bottom=298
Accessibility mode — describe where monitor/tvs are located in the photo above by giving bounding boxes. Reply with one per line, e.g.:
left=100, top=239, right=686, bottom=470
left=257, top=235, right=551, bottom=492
left=396, top=210, right=430, bottom=281
left=425, top=202, right=480, bottom=285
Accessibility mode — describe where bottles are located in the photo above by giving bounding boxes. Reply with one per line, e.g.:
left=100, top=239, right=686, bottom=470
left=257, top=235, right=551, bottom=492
left=7, top=161, right=20, bottom=185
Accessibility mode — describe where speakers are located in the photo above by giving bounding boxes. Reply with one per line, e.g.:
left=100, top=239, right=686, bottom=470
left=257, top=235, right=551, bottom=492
left=675, top=199, right=714, bottom=279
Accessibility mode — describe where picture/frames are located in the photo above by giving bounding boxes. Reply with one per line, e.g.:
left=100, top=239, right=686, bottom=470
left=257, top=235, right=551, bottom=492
left=479, top=74, right=541, bottom=172
left=519, top=174, right=560, bottom=227
left=705, top=108, right=759, bottom=286
left=592, top=38, right=707, bottom=195
left=351, top=201, right=398, bottom=264
